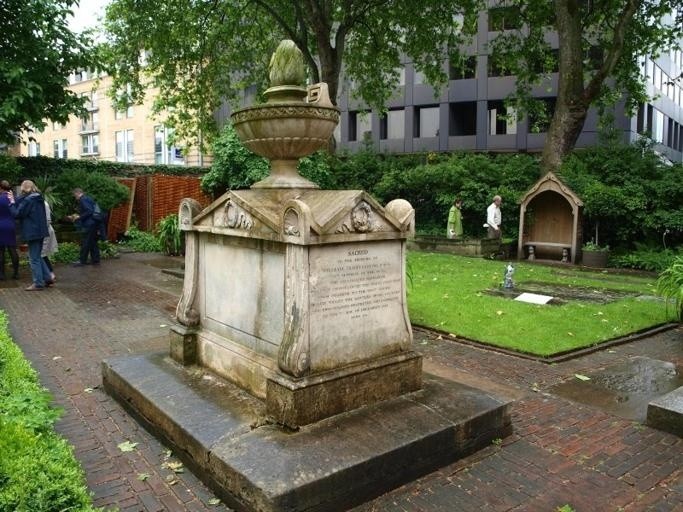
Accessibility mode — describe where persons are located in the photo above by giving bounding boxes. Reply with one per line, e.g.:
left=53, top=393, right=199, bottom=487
left=33, top=189, right=59, bottom=281
left=4, top=179, right=55, bottom=291
left=485, top=195, right=503, bottom=238
left=446, top=198, right=464, bottom=239
left=0, top=179, right=21, bottom=281
left=66, top=187, right=102, bottom=267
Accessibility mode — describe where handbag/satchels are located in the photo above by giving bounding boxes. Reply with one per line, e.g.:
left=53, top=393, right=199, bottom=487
left=93, top=201, right=101, bottom=222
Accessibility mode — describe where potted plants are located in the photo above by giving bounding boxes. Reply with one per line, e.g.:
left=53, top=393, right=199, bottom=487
left=582, top=236, right=609, bottom=269
left=156, top=213, right=186, bottom=257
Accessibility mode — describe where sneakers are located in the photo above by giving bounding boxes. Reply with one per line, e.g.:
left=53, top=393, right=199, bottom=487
left=25, top=283, right=44, bottom=291
left=44, top=272, right=57, bottom=287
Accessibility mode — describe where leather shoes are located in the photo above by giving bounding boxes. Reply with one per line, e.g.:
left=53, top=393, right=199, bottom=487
left=72, top=259, right=80, bottom=264
left=0, top=274, right=20, bottom=280
